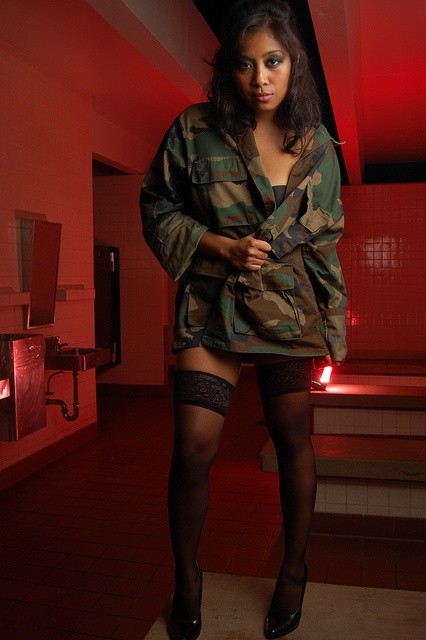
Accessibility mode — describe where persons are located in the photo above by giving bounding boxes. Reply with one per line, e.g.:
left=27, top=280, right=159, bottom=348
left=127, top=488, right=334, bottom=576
left=141, top=0, right=348, bottom=640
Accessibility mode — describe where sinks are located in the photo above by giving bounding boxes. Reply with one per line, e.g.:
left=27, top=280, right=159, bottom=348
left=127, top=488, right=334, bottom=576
left=44, top=346, right=116, bottom=371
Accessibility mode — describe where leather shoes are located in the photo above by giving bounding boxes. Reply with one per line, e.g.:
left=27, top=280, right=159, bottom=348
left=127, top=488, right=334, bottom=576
left=170, top=561, right=203, bottom=640
left=265, top=562, right=307, bottom=639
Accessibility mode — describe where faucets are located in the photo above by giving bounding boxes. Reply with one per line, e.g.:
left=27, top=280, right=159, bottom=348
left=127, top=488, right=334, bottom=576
left=54, top=336, right=70, bottom=350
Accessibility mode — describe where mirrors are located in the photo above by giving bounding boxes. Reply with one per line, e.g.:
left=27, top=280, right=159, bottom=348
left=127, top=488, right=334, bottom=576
left=25, top=219, right=62, bottom=328
